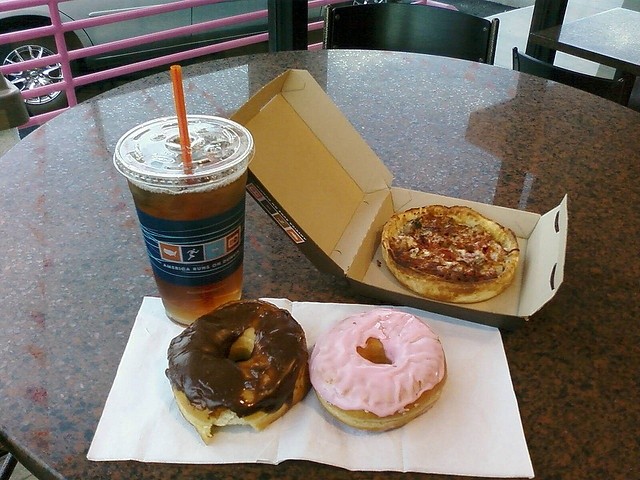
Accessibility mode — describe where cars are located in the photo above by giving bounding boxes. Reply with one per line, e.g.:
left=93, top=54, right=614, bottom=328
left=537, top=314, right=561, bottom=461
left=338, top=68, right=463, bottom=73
left=0, top=0, right=326, bottom=116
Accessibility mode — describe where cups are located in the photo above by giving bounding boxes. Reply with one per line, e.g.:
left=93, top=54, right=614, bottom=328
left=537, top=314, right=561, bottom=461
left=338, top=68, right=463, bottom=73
left=112, top=114, right=256, bottom=327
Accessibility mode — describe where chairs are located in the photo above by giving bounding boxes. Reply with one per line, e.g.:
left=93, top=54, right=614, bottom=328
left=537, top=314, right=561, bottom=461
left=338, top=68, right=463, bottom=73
left=323, top=1, right=500, bottom=65
left=512, top=46, right=625, bottom=103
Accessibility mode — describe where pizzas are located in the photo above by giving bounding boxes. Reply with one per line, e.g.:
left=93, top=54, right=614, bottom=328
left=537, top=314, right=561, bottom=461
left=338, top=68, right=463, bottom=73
left=382, top=204, right=519, bottom=302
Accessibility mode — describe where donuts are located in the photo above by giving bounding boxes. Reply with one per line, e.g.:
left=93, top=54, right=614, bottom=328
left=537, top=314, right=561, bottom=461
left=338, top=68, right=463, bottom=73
left=167, top=300, right=310, bottom=444
left=310, top=307, right=448, bottom=430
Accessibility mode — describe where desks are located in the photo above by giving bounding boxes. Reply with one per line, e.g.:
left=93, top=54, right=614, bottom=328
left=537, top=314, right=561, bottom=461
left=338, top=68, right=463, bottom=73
left=529, top=6, right=640, bottom=75
